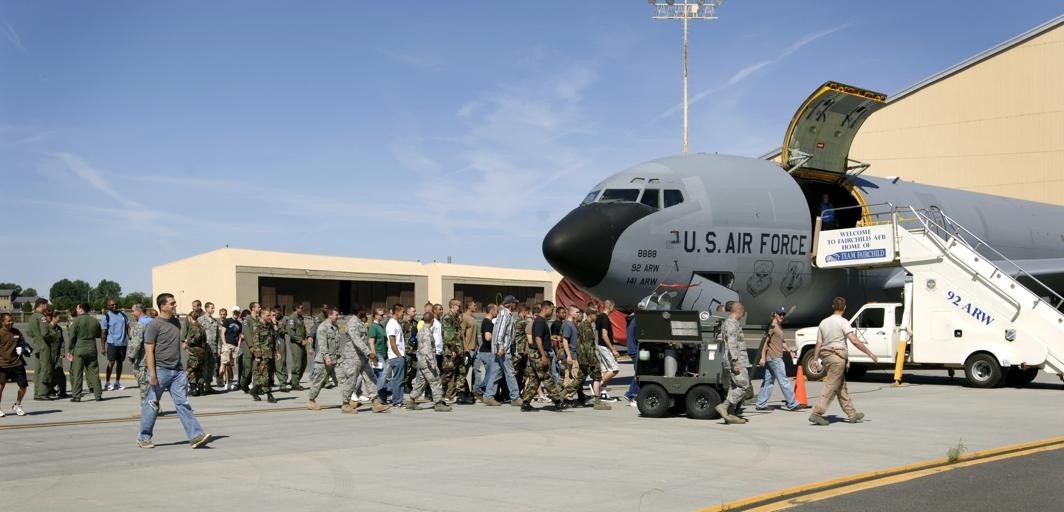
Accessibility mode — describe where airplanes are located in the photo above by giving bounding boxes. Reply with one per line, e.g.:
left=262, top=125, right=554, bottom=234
left=538, top=76, right=1064, bottom=352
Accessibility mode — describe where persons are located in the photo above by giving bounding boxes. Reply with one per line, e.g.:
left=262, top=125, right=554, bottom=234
left=725, top=302, right=753, bottom=421
left=819, top=193, right=835, bottom=228
left=808, top=296, right=880, bottom=425
left=198, top=298, right=343, bottom=410
left=714, top=301, right=745, bottom=425
left=335, top=295, right=642, bottom=412
left=715, top=304, right=726, bottom=313
left=0, top=292, right=214, bottom=449
left=755, top=305, right=806, bottom=411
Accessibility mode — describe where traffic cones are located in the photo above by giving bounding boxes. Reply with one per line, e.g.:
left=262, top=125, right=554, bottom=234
left=790, top=363, right=811, bottom=412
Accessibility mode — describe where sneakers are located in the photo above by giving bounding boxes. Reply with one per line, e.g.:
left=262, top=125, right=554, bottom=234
left=850, top=413, right=864, bottom=422
left=0, top=408, right=5, bottom=417
left=12, top=403, right=25, bottom=415
left=715, top=404, right=746, bottom=425
left=791, top=404, right=806, bottom=412
left=307, top=398, right=321, bottom=410
left=137, top=439, right=155, bottom=448
left=624, top=395, right=637, bottom=407
left=191, top=433, right=211, bottom=448
left=104, top=384, right=116, bottom=391
left=113, top=383, right=124, bottom=390
left=34, top=389, right=102, bottom=402
left=225, top=380, right=304, bottom=402
left=808, top=414, right=830, bottom=425
left=757, top=406, right=774, bottom=410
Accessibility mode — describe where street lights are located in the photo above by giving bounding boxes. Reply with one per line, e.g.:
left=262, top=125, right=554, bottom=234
left=645, top=0, right=722, bottom=154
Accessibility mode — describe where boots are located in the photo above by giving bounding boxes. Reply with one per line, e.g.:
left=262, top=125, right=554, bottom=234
left=341, top=384, right=618, bottom=413
left=189, top=383, right=217, bottom=396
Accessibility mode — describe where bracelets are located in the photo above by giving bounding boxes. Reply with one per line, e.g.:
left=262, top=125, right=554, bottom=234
left=788, top=350, right=792, bottom=353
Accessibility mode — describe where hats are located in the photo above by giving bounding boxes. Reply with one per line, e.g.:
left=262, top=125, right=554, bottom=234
left=775, top=304, right=786, bottom=315
left=504, top=295, right=519, bottom=303
left=231, top=306, right=241, bottom=312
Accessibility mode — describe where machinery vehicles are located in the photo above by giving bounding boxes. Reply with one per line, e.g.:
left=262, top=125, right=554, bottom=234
left=796, top=201, right=1064, bottom=390
